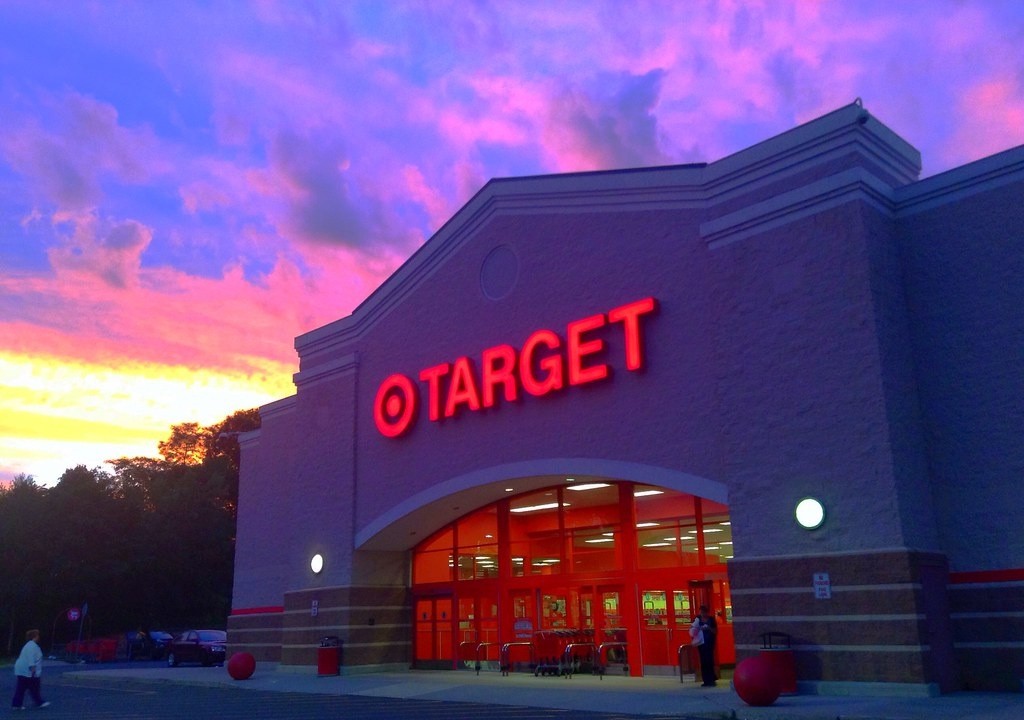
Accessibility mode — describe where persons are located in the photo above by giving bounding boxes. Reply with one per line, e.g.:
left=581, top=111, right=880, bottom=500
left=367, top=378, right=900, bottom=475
left=692, top=605, right=717, bottom=688
left=125, top=629, right=137, bottom=663
left=12, top=629, right=50, bottom=711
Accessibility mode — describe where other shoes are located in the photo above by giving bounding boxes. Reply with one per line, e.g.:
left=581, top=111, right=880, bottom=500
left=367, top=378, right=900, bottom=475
left=700, top=682, right=717, bottom=687
left=12, top=706, right=26, bottom=712
left=38, top=702, right=50, bottom=708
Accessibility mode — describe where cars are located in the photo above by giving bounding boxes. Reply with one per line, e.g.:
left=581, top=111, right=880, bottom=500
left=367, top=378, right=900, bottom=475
left=135, top=618, right=190, bottom=658
left=162, top=630, right=228, bottom=665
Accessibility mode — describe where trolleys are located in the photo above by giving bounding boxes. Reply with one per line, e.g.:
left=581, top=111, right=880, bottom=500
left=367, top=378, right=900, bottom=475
left=532, top=627, right=626, bottom=680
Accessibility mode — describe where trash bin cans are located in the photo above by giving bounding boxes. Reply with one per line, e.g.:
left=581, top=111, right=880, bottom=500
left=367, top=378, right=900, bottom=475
left=758, top=631, right=798, bottom=698
left=315, top=635, right=340, bottom=677
